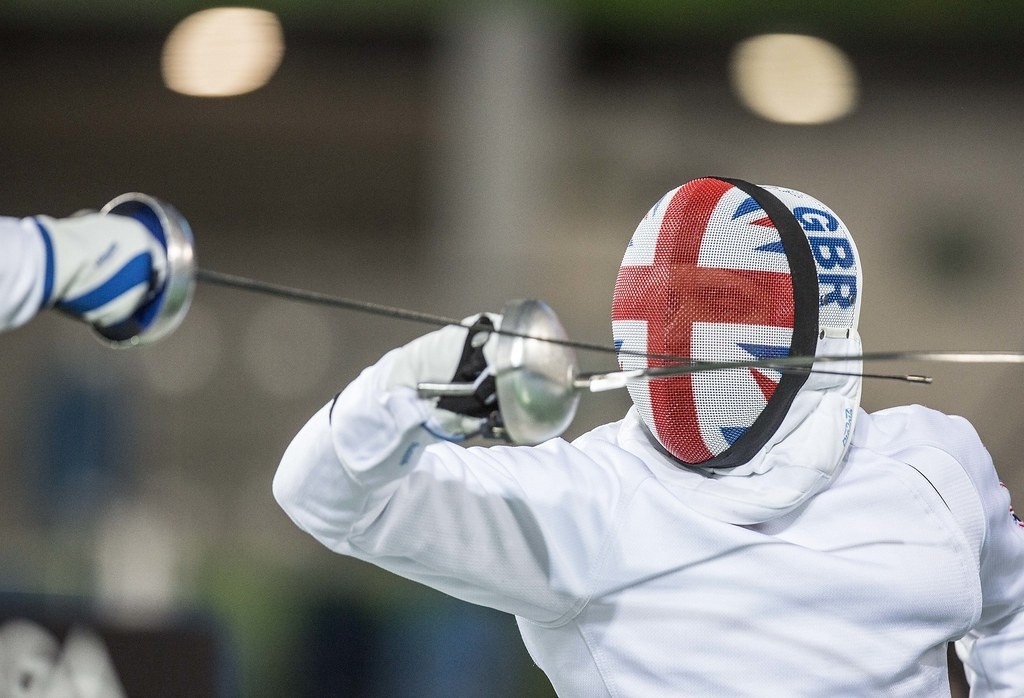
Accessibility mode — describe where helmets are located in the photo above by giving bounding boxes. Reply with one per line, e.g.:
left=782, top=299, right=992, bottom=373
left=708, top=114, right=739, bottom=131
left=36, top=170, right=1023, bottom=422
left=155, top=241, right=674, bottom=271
left=611, top=176, right=862, bottom=525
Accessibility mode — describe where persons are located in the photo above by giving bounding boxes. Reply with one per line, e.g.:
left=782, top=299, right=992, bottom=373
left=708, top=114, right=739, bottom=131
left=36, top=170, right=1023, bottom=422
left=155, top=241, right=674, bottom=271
left=0, top=210, right=171, bottom=347
left=271, top=177, right=1024, bottom=697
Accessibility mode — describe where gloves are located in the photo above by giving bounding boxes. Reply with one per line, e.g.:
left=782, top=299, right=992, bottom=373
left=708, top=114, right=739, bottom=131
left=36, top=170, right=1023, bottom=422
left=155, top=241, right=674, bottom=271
left=30, top=209, right=165, bottom=329
left=329, top=311, right=504, bottom=490
